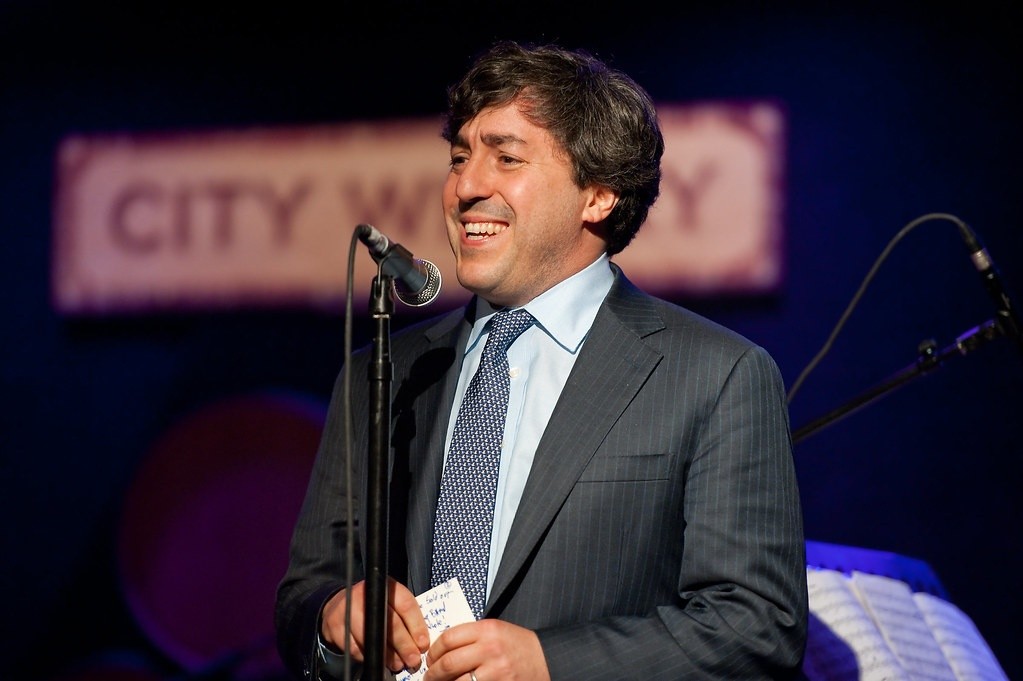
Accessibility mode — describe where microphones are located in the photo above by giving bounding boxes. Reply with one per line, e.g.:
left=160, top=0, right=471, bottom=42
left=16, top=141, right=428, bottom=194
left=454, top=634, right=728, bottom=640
left=963, top=229, right=1023, bottom=358
left=355, top=224, right=442, bottom=307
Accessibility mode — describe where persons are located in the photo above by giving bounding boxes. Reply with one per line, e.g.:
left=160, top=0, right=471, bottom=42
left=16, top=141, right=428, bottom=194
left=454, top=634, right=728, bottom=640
left=275, top=43, right=809, bottom=681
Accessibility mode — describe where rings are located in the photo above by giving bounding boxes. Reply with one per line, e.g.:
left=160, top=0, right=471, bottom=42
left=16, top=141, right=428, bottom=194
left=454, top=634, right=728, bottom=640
left=470, top=670, right=477, bottom=681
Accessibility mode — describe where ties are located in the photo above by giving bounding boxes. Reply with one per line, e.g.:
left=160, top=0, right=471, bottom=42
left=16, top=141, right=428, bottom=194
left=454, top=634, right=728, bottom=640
left=430, top=308, right=539, bottom=621
